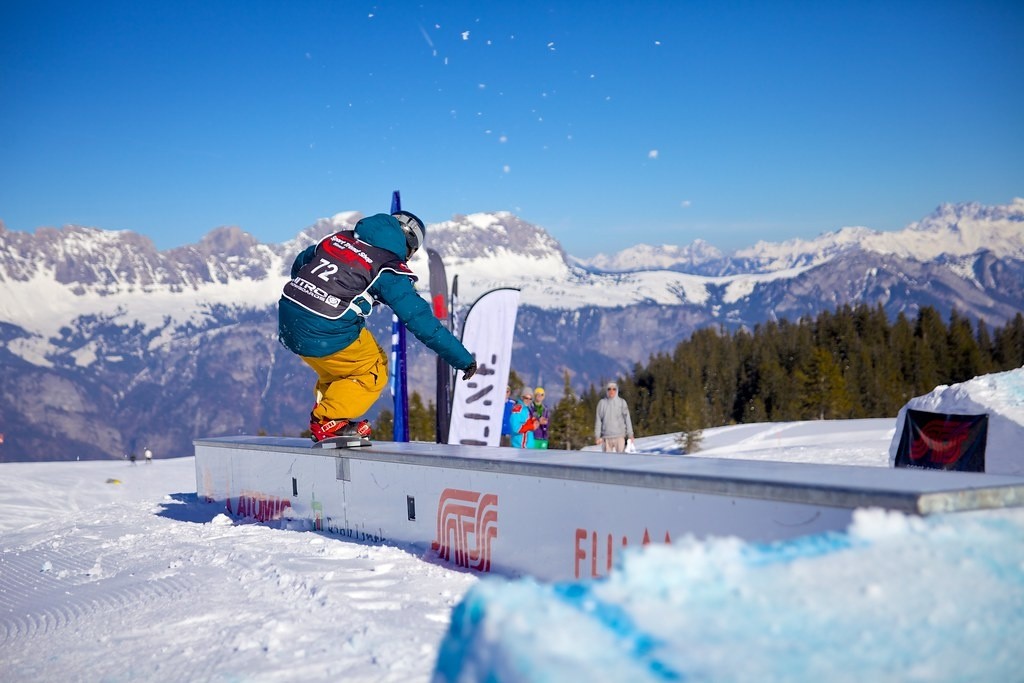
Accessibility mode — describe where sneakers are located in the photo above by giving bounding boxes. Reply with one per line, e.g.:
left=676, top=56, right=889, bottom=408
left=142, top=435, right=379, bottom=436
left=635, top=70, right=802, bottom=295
left=310, top=420, right=369, bottom=442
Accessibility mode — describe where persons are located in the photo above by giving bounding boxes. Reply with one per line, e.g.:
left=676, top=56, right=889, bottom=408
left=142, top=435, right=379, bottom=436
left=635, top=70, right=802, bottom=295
left=501, top=385, right=516, bottom=446
left=278, top=211, right=477, bottom=443
left=509, top=387, right=539, bottom=448
left=594, top=382, right=635, bottom=452
left=531, top=387, right=551, bottom=449
left=144, top=449, right=152, bottom=462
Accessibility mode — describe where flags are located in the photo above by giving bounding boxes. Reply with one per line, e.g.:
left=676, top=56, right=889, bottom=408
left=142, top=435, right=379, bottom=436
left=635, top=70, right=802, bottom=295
left=390, top=311, right=410, bottom=443
left=449, top=288, right=522, bottom=445
left=425, top=248, right=449, bottom=445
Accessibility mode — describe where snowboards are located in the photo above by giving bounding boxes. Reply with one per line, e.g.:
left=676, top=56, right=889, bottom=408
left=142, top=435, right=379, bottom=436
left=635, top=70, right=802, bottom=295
left=311, top=435, right=372, bottom=449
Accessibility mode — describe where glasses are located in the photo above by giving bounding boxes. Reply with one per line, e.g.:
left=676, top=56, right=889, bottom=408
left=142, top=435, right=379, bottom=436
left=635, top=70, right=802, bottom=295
left=607, top=388, right=617, bottom=390
left=507, top=391, right=509, bottom=393
left=523, top=396, right=533, bottom=400
left=536, top=392, right=544, bottom=396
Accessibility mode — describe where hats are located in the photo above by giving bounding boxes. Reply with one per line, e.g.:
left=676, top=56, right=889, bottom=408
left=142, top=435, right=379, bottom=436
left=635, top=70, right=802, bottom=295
left=521, top=387, right=533, bottom=397
left=534, top=387, right=545, bottom=394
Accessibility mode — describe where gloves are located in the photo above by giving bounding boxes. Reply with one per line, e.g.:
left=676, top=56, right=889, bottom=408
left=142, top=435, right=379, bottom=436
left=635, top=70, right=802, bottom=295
left=462, top=360, right=476, bottom=380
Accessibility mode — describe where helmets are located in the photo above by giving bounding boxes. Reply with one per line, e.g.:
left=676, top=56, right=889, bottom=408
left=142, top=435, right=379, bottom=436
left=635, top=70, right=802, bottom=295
left=390, top=211, right=426, bottom=249
